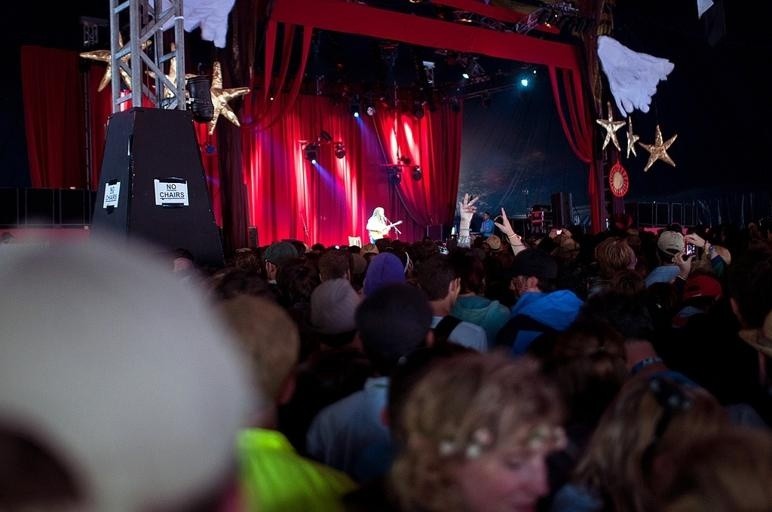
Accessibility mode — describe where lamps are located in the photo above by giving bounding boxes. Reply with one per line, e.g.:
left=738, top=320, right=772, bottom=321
left=304, top=93, right=492, bottom=166
left=185, top=75, right=214, bottom=123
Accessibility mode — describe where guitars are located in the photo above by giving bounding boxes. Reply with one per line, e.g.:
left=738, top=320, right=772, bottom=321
left=371, top=220, right=402, bottom=240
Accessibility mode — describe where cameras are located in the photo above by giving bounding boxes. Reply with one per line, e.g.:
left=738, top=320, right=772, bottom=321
left=683, top=239, right=697, bottom=256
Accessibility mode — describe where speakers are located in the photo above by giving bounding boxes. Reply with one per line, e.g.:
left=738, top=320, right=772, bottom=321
left=551, top=191, right=573, bottom=229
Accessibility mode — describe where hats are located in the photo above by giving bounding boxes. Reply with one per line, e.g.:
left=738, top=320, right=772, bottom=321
left=483, top=235, right=501, bottom=250
left=657, top=230, right=686, bottom=256
left=738, top=312, right=772, bottom=356
left=682, top=273, right=721, bottom=299
left=309, top=278, right=362, bottom=333
left=363, top=252, right=406, bottom=296
